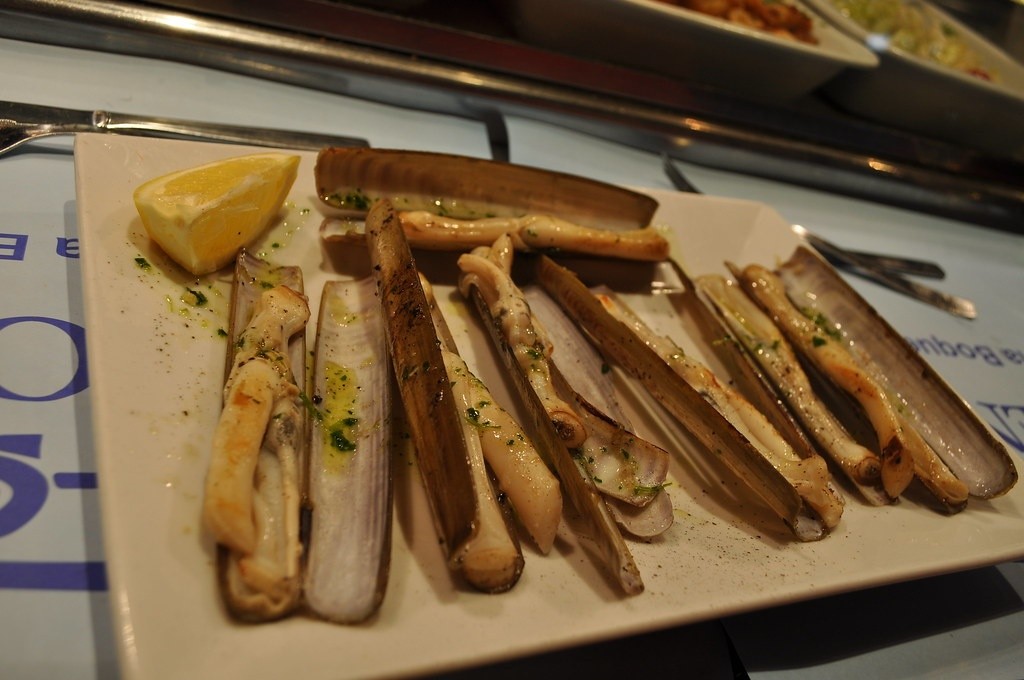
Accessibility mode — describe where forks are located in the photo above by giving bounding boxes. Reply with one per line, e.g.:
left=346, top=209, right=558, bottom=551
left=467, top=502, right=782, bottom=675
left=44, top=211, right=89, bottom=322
left=660, top=148, right=977, bottom=320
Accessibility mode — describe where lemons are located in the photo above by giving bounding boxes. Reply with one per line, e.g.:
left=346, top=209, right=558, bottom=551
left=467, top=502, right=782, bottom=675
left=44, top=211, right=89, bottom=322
left=132, top=151, right=302, bottom=275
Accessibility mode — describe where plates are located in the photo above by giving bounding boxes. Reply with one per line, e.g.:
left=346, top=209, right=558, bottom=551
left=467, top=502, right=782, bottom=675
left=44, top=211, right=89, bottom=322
left=72, top=131, right=1023, bottom=680
